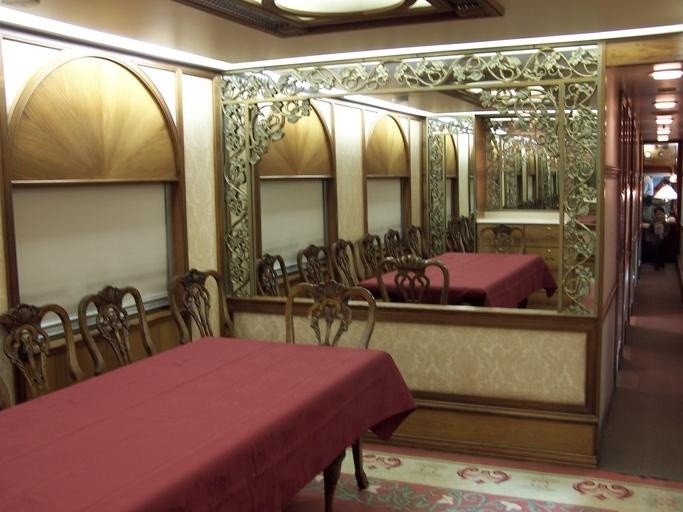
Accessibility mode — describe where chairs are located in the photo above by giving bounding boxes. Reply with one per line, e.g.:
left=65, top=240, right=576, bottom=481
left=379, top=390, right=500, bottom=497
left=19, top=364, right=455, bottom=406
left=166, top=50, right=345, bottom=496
left=168, top=268, right=237, bottom=346
left=284, top=279, right=377, bottom=488
left=0, top=303, right=86, bottom=408
left=78, top=285, right=157, bottom=376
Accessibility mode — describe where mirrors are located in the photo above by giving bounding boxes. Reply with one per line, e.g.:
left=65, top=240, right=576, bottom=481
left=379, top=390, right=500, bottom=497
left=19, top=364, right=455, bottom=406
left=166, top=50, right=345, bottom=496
left=221, top=41, right=605, bottom=319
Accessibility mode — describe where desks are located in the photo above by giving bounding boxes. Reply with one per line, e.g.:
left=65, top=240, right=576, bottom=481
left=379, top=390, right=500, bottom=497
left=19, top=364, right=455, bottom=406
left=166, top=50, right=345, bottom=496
left=1, top=335, right=417, bottom=512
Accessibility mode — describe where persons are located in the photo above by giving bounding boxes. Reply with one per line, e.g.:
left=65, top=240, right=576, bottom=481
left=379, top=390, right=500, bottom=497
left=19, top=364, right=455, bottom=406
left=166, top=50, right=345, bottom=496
left=646, top=207, right=671, bottom=271
left=665, top=211, right=675, bottom=222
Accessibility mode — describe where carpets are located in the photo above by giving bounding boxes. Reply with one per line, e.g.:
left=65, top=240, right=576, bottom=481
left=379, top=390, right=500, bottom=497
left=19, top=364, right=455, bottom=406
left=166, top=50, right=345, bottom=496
left=279, top=442, right=683, bottom=512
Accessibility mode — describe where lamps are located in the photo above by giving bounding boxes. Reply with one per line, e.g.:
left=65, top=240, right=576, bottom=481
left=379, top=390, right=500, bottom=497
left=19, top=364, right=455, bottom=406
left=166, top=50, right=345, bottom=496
left=653, top=184, right=678, bottom=217
